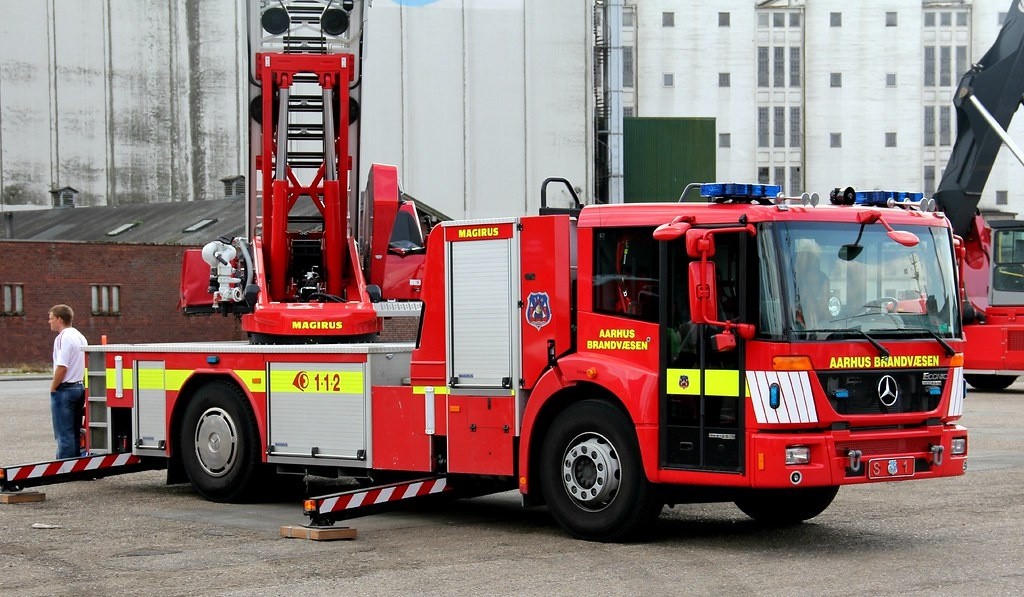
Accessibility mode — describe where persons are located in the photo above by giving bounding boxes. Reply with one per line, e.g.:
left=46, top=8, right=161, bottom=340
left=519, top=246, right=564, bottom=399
left=670, top=318, right=697, bottom=365
left=48, top=304, right=87, bottom=458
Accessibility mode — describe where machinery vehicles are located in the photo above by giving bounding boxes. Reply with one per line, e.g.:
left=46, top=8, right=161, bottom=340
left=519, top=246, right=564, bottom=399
left=2, top=0, right=969, bottom=542
left=952, top=1, right=1024, bottom=395
